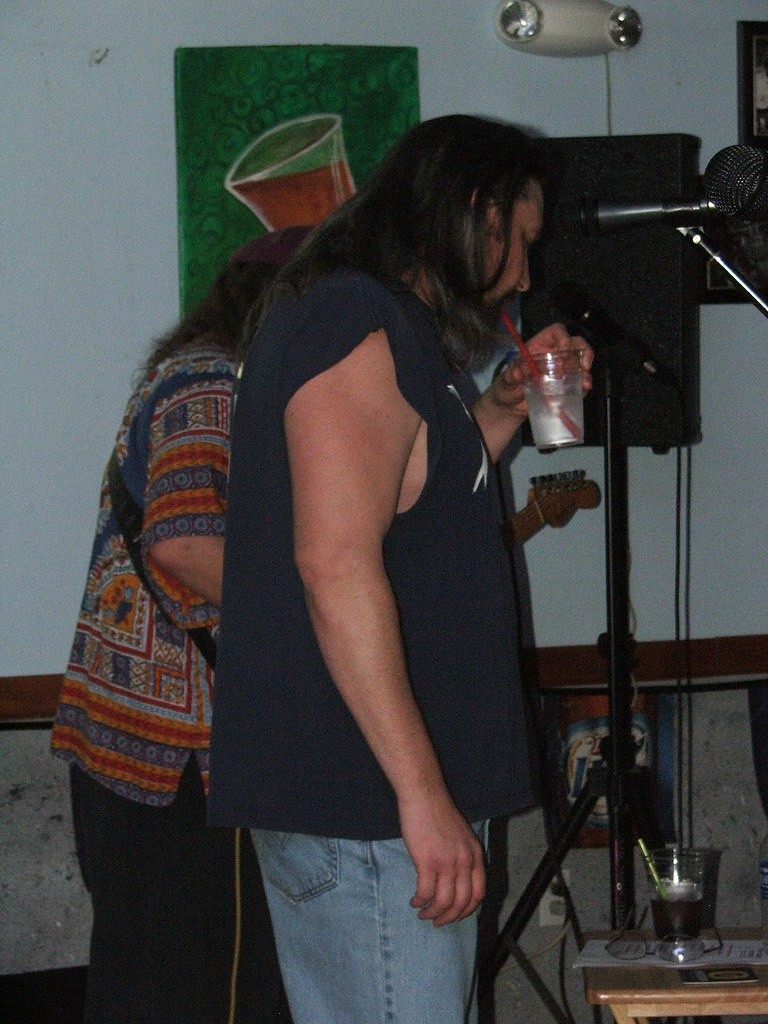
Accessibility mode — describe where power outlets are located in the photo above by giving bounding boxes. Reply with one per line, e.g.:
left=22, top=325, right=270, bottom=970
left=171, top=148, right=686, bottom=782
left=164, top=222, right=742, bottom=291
left=539, top=866, right=575, bottom=927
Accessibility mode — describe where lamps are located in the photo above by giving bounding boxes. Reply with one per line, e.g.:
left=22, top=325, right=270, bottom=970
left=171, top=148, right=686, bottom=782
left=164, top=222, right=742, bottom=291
left=499, top=1, right=644, bottom=62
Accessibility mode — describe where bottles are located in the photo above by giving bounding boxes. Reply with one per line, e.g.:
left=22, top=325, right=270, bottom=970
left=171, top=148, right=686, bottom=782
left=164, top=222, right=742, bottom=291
left=758, top=833, right=768, bottom=937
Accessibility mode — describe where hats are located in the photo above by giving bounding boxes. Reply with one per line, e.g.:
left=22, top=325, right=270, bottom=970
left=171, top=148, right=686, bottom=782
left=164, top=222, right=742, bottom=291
left=231, top=225, right=314, bottom=262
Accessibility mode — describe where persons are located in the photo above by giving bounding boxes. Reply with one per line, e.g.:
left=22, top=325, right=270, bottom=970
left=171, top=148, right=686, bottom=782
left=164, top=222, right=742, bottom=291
left=50, top=225, right=317, bottom=1024
left=206, top=114, right=594, bottom=1024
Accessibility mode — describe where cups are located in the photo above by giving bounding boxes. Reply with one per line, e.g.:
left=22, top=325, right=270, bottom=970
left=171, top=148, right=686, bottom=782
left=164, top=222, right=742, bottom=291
left=641, top=848, right=723, bottom=942
left=513, top=348, right=586, bottom=450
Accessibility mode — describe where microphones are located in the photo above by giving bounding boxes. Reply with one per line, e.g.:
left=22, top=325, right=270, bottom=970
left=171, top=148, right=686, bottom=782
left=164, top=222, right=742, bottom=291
left=579, top=195, right=719, bottom=236
left=556, top=289, right=666, bottom=384
left=706, top=145, right=768, bottom=225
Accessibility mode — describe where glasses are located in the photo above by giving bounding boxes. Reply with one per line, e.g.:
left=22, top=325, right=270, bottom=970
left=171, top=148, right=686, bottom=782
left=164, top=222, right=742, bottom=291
left=604, top=905, right=724, bottom=964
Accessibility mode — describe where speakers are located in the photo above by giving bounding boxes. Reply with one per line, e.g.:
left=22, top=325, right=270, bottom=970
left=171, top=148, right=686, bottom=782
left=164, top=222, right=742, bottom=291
left=518, top=135, right=706, bottom=454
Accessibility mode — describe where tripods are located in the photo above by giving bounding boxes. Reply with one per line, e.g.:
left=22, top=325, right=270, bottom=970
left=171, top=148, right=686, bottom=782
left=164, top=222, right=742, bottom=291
left=474, top=368, right=669, bottom=1024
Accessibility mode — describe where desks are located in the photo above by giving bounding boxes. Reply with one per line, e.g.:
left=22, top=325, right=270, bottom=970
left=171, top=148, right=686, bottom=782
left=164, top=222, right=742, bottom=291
left=578, top=927, right=768, bottom=1024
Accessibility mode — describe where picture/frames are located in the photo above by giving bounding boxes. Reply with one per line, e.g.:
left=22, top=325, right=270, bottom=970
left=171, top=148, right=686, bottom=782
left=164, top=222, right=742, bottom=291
left=737, top=22, right=768, bottom=145
